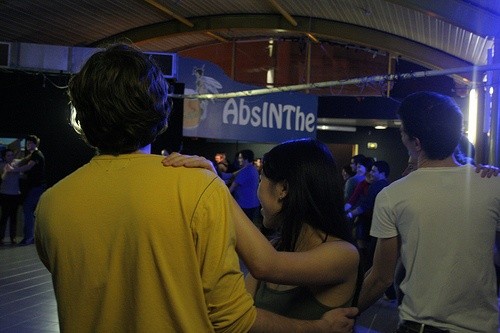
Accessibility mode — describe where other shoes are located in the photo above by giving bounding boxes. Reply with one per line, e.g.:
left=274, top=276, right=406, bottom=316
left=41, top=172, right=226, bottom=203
left=20, top=239, right=35, bottom=246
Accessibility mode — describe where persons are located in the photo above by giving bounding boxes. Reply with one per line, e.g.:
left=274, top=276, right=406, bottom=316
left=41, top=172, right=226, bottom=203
left=0, top=149, right=29, bottom=243
left=365, top=135, right=500, bottom=277
left=6, top=135, right=44, bottom=245
left=358, top=91, right=500, bottom=333
left=337, top=149, right=385, bottom=247
left=215, top=151, right=263, bottom=229
left=161, top=135, right=360, bottom=332
left=35, top=45, right=360, bottom=333
